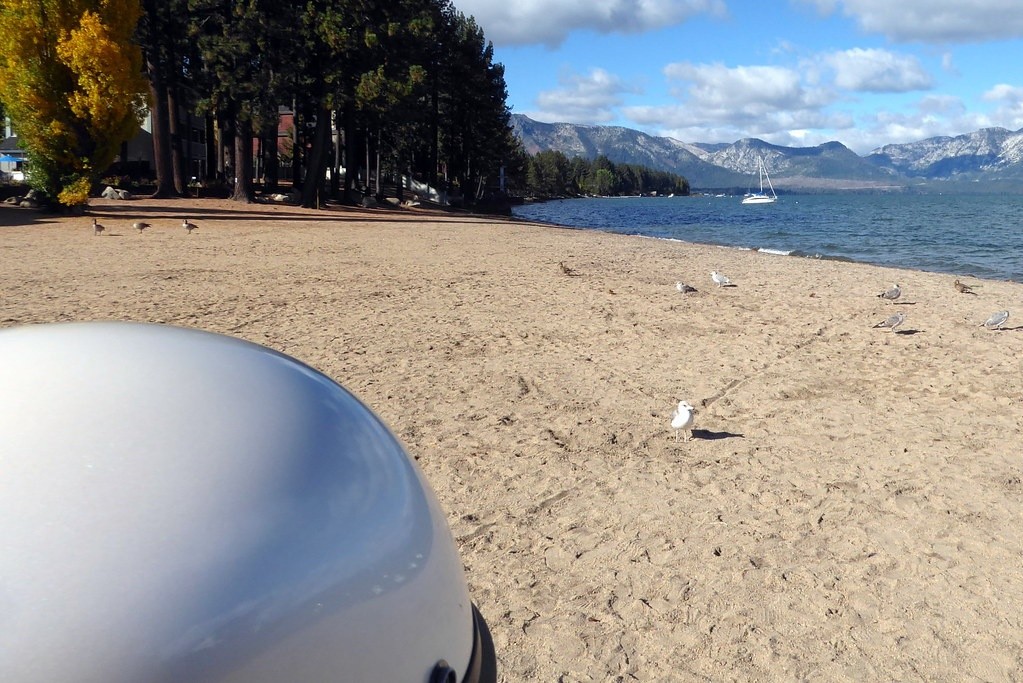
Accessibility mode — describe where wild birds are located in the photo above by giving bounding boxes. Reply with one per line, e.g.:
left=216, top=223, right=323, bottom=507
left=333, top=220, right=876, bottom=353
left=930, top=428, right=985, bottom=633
left=92, top=217, right=106, bottom=236
left=876, top=284, right=901, bottom=306
left=676, top=281, right=698, bottom=296
left=953, top=279, right=976, bottom=295
left=556, top=262, right=572, bottom=276
left=133, top=222, right=152, bottom=235
left=182, top=219, right=198, bottom=234
left=709, top=270, right=733, bottom=288
left=872, top=311, right=907, bottom=334
left=669, top=400, right=695, bottom=443
left=979, top=310, right=1009, bottom=331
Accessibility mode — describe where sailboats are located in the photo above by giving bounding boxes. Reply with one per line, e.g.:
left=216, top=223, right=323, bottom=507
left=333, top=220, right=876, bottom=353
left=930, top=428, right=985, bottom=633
left=742, top=155, right=778, bottom=204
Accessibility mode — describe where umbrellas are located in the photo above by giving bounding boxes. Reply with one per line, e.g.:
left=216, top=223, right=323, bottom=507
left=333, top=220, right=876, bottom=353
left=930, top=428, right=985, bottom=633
left=0, top=154, right=29, bottom=174
